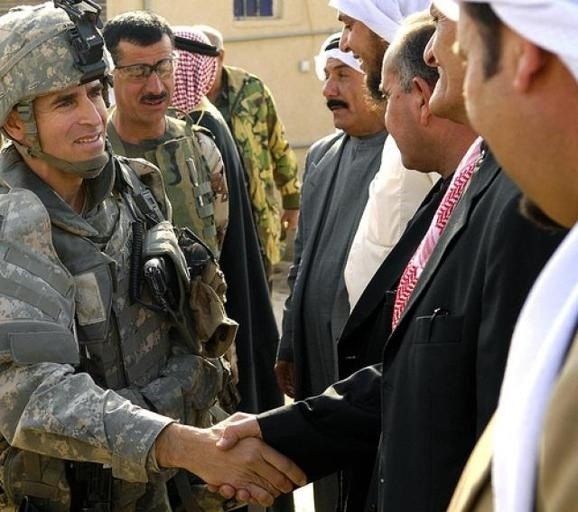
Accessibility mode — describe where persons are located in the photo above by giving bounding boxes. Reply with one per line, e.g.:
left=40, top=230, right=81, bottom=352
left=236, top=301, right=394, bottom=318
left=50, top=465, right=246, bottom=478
left=2, top=0, right=578, bottom=512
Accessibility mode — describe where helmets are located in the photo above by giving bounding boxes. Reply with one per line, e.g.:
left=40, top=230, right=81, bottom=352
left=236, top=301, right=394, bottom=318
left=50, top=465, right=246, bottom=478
left=0, top=0, right=115, bottom=128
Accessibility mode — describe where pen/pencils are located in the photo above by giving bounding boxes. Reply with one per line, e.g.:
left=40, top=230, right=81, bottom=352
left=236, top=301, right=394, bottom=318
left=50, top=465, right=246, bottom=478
left=188, top=158, right=205, bottom=209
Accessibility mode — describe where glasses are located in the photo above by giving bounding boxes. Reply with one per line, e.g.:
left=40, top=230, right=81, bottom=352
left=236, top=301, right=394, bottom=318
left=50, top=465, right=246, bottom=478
left=115, top=53, right=178, bottom=80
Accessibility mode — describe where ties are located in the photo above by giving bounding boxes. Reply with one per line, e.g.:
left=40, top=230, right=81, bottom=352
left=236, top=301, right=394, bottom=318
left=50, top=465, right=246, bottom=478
left=391, top=153, right=485, bottom=339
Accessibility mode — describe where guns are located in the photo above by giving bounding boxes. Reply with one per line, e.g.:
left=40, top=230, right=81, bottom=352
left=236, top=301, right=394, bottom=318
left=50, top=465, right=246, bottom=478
left=135, top=189, right=242, bottom=509
